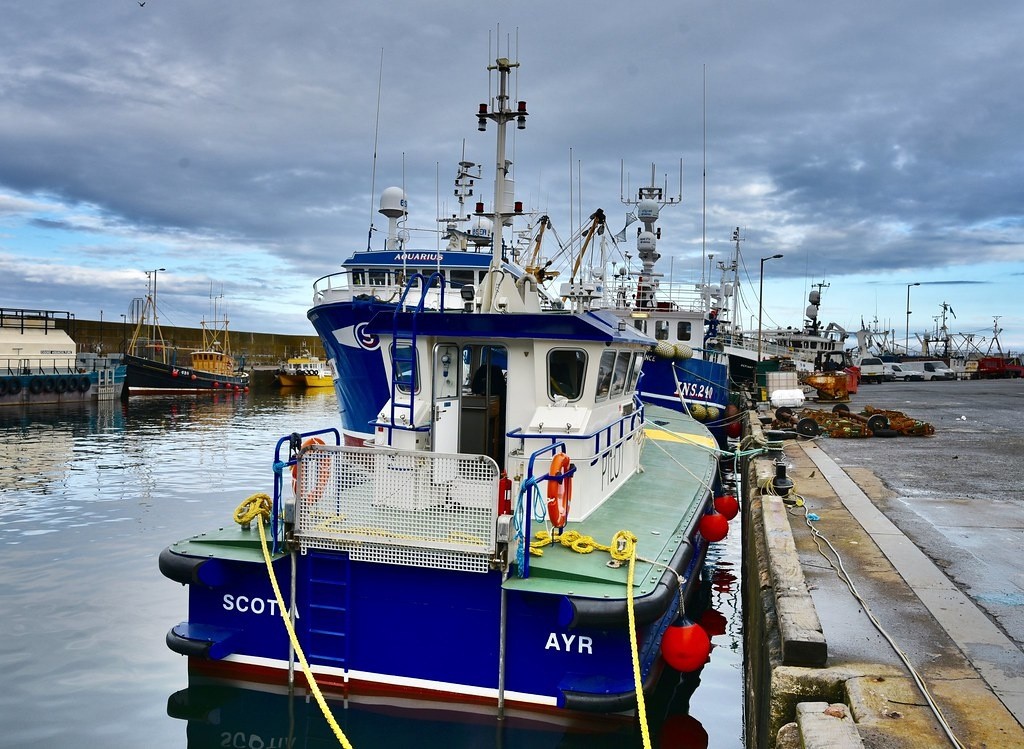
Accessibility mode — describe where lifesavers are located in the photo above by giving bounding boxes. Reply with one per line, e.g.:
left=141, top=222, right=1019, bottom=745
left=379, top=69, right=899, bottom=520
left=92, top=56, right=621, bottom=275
left=292, top=438, right=329, bottom=503
left=549, top=453, right=573, bottom=526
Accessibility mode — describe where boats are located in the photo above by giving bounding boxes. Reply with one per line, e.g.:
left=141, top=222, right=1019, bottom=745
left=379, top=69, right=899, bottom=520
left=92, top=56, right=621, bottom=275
left=0, top=136, right=1024, bottom=451
left=157, top=21, right=735, bottom=740
